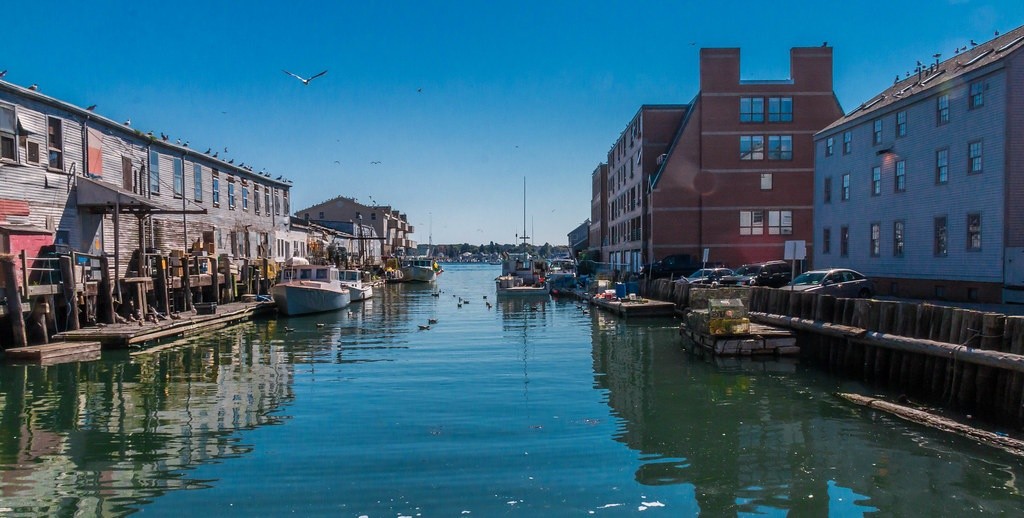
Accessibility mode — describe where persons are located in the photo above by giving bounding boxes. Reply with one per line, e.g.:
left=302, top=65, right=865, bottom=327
left=335, top=251, right=341, bottom=268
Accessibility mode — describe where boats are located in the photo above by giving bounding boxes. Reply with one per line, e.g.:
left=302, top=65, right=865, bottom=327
left=271, top=257, right=351, bottom=316
left=679, top=298, right=802, bottom=357
left=400, top=260, right=437, bottom=283
left=544, top=252, right=575, bottom=274
left=338, top=270, right=373, bottom=301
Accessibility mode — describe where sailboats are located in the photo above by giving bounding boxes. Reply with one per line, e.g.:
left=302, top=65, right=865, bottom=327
left=494, top=176, right=551, bottom=296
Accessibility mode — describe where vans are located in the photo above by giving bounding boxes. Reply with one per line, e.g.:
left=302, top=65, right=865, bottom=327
left=728, top=261, right=793, bottom=288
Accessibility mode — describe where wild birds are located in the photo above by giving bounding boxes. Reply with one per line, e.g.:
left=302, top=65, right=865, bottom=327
left=278, top=69, right=330, bottom=86
left=453, top=294, right=456, bottom=297
left=284, top=326, right=296, bottom=331
left=575, top=305, right=582, bottom=309
left=114, top=304, right=182, bottom=327
left=95, top=322, right=107, bottom=331
left=347, top=311, right=353, bottom=315
left=431, top=289, right=445, bottom=296
left=582, top=310, right=588, bottom=314
left=191, top=304, right=197, bottom=315
left=315, top=322, right=325, bottom=327
left=300, top=280, right=305, bottom=286
left=485, top=301, right=492, bottom=308
left=483, top=295, right=487, bottom=298
left=458, top=297, right=470, bottom=304
left=417, top=324, right=432, bottom=330
left=0, top=69, right=427, bottom=185
left=311, top=194, right=376, bottom=206
left=457, top=303, right=463, bottom=308
left=426, top=318, right=438, bottom=322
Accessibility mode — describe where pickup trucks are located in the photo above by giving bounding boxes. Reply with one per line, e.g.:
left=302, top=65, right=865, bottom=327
left=637, top=254, right=725, bottom=279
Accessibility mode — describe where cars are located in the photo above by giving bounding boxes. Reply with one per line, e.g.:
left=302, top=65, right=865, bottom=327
left=675, top=267, right=742, bottom=286
left=778, top=268, right=877, bottom=299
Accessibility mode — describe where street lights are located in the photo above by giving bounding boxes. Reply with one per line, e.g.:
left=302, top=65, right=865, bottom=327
left=359, top=214, right=365, bottom=265
